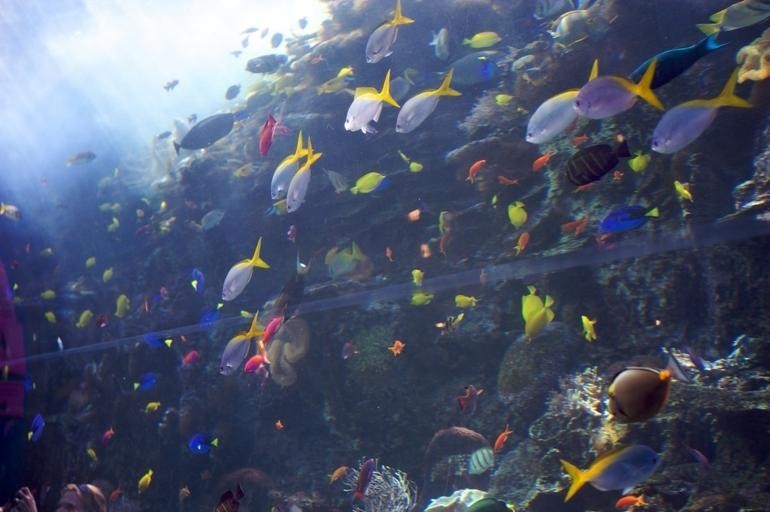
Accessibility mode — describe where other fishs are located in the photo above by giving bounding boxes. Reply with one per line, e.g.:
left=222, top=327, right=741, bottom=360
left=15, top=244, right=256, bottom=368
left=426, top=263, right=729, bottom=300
left=0, top=0, right=769, bottom=511
